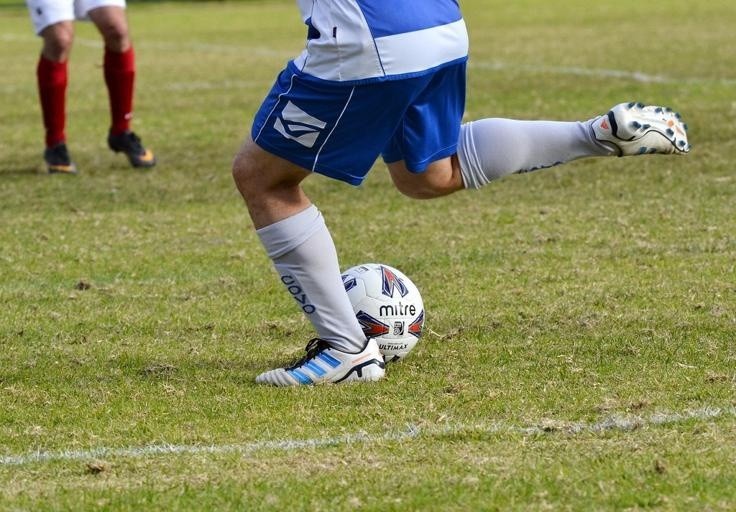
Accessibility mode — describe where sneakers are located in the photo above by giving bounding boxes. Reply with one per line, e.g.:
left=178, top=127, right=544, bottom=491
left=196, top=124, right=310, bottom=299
left=108, top=128, right=156, bottom=170
left=592, top=100, right=691, bottom=159
left=254, top=334, right=387, bottom=389
left=43, top=144, right=78, bottom=174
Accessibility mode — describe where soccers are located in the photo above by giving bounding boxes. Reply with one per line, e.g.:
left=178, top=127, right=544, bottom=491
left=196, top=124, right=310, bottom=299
left=341, top=262, right=425, bottom=365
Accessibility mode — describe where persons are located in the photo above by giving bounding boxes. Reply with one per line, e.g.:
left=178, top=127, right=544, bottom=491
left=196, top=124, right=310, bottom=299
left=17, top=1, right=158, bottom=178
left=230, top=0, right=690, bottom=389
